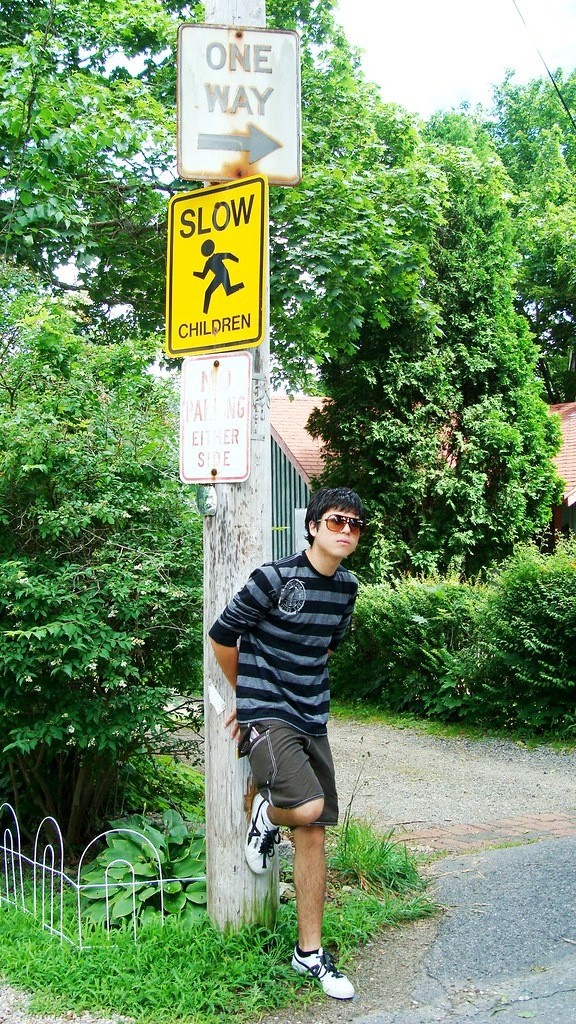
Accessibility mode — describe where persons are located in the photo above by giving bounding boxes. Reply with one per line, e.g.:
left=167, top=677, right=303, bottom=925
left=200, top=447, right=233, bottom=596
left=209, top=487, right=366, bottom=1000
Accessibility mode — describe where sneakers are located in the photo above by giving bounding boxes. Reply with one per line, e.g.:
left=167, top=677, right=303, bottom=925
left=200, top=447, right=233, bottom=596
left=291, top=945, right=355, bottom=999
left=244, top=792, right=281, bottom=874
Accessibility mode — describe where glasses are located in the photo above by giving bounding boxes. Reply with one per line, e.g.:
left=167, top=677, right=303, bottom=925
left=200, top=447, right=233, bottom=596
left=319, top=513, right=361, bottom=532
